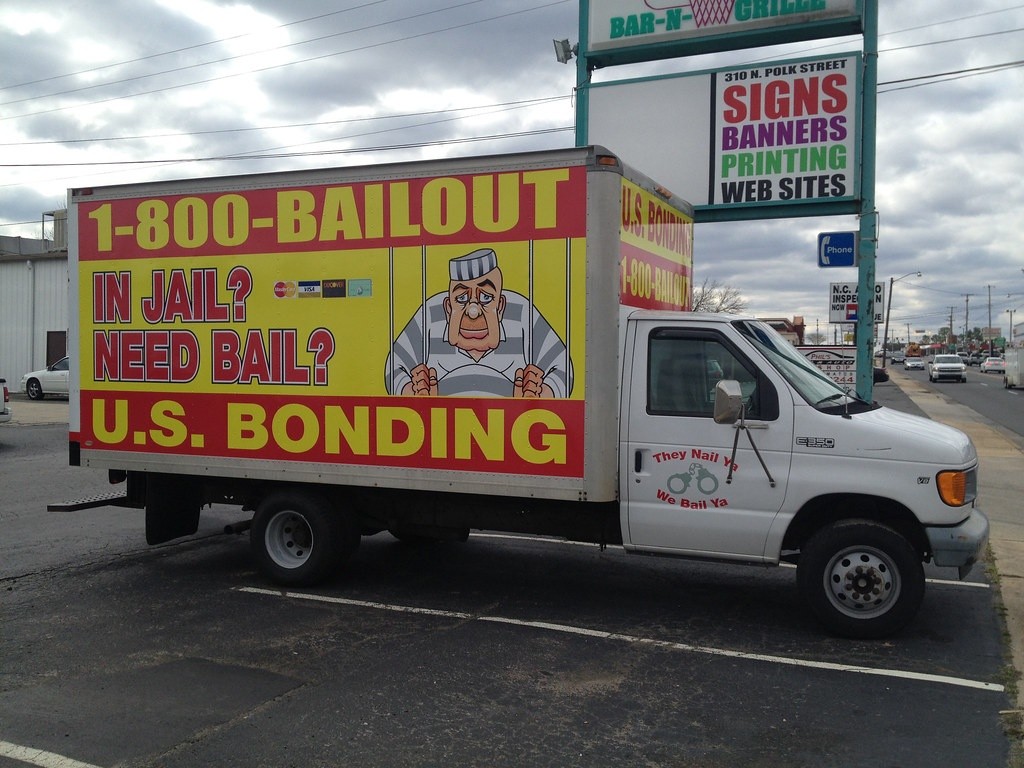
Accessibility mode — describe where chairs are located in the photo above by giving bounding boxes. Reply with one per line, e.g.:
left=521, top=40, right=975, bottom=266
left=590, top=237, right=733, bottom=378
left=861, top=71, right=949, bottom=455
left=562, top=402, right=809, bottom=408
left=658, top=359, right=700, bottom=412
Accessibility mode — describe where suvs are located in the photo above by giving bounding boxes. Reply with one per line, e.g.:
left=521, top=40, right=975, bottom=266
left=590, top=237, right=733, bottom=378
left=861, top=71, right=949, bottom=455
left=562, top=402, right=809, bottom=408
left=62, top=143, right=989, bottom=639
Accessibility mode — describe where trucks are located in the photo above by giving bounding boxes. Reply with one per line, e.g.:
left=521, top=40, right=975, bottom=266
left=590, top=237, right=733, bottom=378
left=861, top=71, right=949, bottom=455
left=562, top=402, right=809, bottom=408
left=1003, top=345, right=1024, bottom=390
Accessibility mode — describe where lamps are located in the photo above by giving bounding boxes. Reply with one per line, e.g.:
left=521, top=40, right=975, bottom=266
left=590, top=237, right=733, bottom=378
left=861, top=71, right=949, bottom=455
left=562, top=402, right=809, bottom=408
left=553, top=37, right=579, bottom=64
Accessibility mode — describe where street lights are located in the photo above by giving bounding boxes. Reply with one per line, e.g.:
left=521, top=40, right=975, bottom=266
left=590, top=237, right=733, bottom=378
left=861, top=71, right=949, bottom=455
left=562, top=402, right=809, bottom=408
left=881, top=271, right=922, bottom=368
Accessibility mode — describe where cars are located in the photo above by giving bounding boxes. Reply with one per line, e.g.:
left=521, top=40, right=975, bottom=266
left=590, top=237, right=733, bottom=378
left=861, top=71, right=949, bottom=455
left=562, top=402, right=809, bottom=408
left=927, top=353, right=969, bottom=383
left=21, top=356, right=68, bottom=400
left=956, top=352, right=969, bottom=364
left=903, top=357, right=925, bottom=372
left=980, top=356, right=1005, bottom=373
left=874, top=365, right=891, bottom=384
left=875, top=349, right=890, bottom=358
left=970, top=353, right=985, bottom=366
left=891, top=352, right=907, bottom=365
